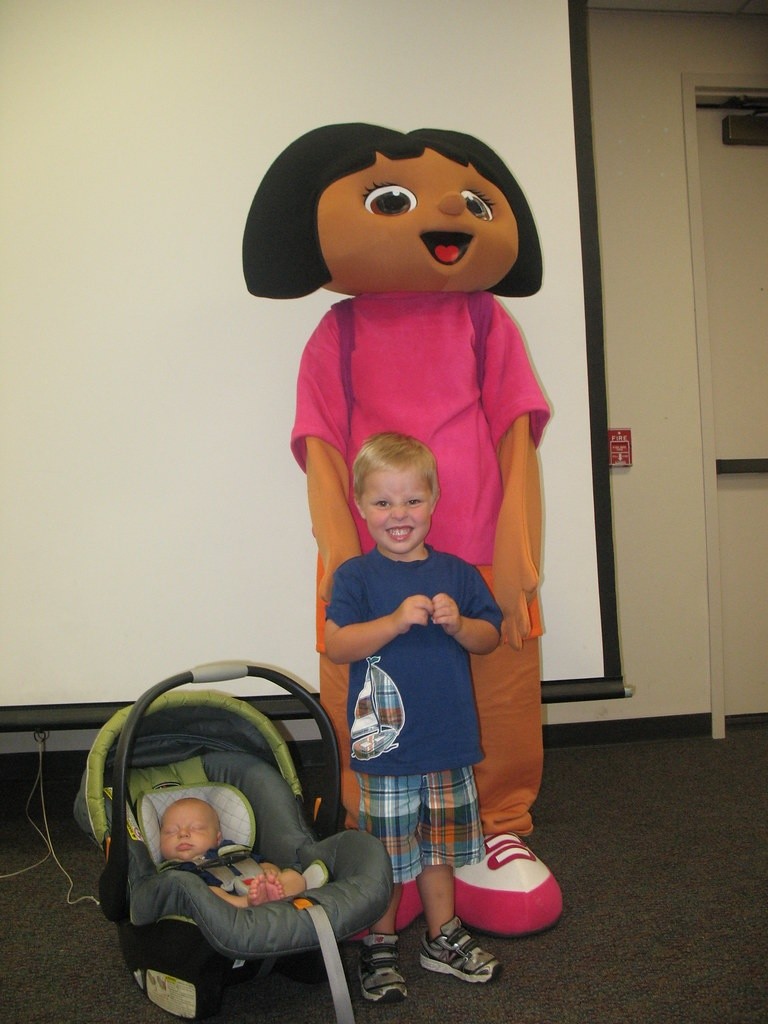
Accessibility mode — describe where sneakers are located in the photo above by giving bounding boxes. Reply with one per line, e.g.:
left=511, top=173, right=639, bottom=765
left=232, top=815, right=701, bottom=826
left=419, top=918, right=502, bottom=984
left=358, top=934, right=407, bottom=1002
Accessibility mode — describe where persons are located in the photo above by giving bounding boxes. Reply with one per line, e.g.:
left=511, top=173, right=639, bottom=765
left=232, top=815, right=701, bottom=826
left=324, top=432, right=503, bottom=1002
left=242, top=123, right=563, bottom=942
left=160, top=798, right=306, bottom=910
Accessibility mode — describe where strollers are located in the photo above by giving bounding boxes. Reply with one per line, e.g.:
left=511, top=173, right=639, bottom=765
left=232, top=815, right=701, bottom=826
left=72, top=657, right=394, bottom=1024
